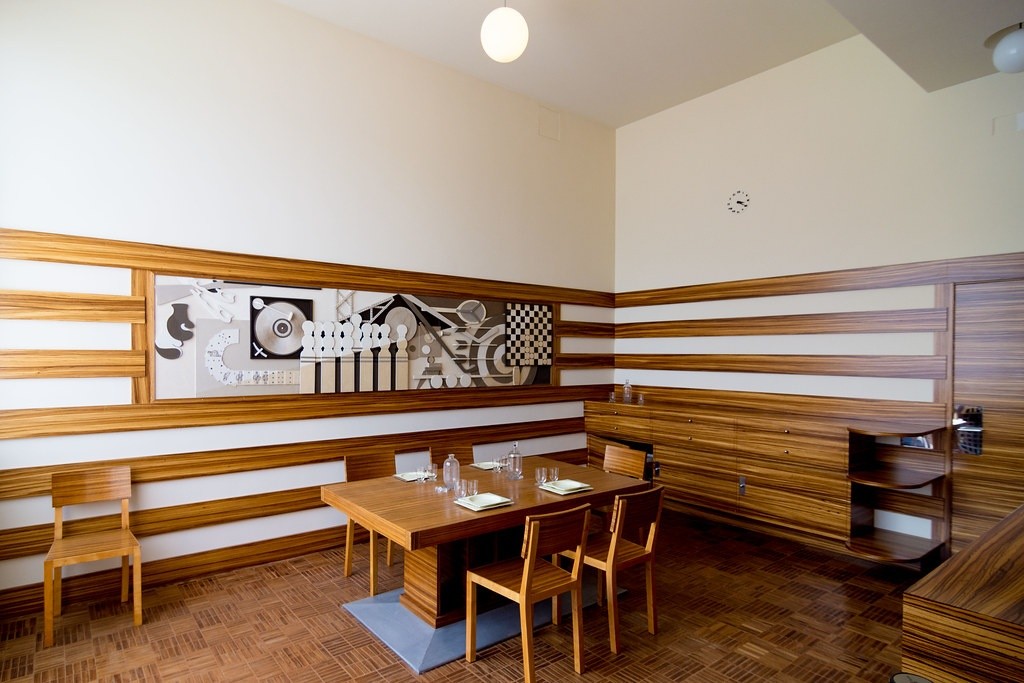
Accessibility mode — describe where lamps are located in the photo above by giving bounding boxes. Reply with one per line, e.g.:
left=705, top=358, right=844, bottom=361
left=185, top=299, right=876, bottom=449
left=992, top=22, right=1024, bottom=74
left=481, top=0, right=529, bottom=63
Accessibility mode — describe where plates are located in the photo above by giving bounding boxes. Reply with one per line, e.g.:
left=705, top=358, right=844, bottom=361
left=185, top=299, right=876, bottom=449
left=393, top=470, right=437, bottom=483
left=453, top=492, right=515, bottom=512
left=469, top=461, right=508, bottom=470
left=545, top=479, right=595, bottom=496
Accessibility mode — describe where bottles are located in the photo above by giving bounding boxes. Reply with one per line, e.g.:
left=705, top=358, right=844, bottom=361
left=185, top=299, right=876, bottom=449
left=443, top=454, right=459, bottom=489
left=623, top=379, right=632, bottom=402
left=506, top=440, right=524, bottom=480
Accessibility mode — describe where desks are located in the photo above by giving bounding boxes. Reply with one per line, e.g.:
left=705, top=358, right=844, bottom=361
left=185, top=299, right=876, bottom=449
left=901, top=503, right=1024, bottom=683
left=320, top=455, right=650, bottom=675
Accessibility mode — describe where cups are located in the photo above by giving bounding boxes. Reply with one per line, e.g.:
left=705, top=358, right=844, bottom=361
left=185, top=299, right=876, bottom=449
left=609, top=392, right=616, bottom=402
left=455, top=479, right=467, bottom=498
left=468, top=479, right=479, bottom=495
left=535, top=467, right=548, bottom=485
left=416, top=467, right=425, bottom=482
left=501, top=456, right=508, bottom=469
left=549, top=467, right=558, bottom=481
left=428, top=464, right=439, bottom=481
left=638, top=394, right=644, bottom=404
left=492, top=457, right=500, bottom=471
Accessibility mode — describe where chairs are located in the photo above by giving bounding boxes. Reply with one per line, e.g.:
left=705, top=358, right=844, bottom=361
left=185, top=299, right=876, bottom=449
left=585, top=447, right=647, bottom=544
left=43, top=464, right=142, bottom=648
left=344, top=447, right=397, bottom=597
left=429, top=441, right=474, bottom=469
left=550, top=484, right=664, bottom=655
left=466, top=504, right=592, bottom=683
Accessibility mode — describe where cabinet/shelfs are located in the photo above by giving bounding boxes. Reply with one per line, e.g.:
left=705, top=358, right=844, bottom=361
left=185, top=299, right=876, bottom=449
left=583, top=400, right=946, bottom=564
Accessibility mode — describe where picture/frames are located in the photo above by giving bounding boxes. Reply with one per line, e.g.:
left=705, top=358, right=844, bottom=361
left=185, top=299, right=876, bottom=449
left=146, top=269, right=558, bottom=404
